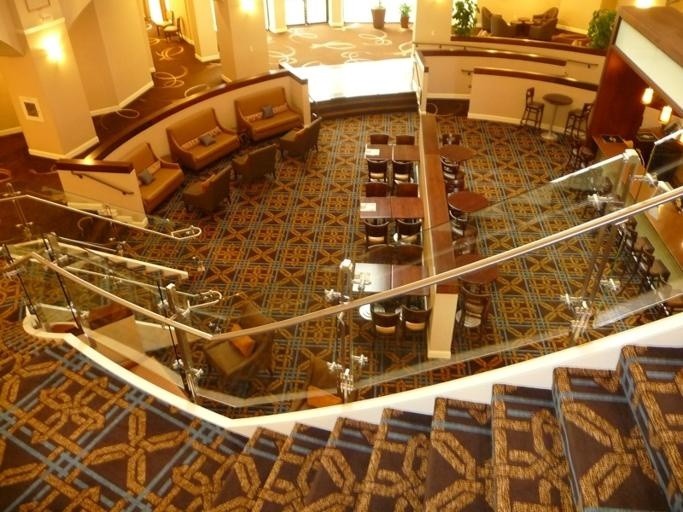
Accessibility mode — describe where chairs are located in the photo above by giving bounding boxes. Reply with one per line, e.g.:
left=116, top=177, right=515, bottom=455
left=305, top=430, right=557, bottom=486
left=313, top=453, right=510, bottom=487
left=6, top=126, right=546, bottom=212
left=289, top=357, right=364, bottom=411
left=519, top=86, right=683, bottom=325
left=279, top=117, right=323, bottom=163
left=482, top=6, right=558, bottom=42
left=203, top=303, right=275, bottom=393
left=157, top=11, right=183, bottom=44
left=352, top=133, right=499, bottom=351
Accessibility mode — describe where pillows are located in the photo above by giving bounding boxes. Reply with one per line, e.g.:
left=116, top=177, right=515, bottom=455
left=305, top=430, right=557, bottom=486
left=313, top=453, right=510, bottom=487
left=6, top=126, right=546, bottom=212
left=229, top=324, right=256, bottom=357
left=308, top=385, right=343, bottom=408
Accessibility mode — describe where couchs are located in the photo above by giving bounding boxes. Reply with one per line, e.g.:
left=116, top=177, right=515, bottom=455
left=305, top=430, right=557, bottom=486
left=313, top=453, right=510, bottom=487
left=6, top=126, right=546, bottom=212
left=121, top=87, right=303, bottom=216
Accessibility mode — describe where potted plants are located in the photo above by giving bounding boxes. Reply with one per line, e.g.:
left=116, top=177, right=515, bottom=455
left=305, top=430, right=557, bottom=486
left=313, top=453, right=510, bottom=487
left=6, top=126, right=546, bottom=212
left=399, top=3, right=411, bottom=28
left=371, top=0, right=385, bottom=28
